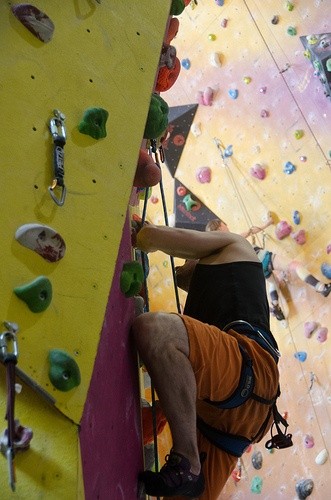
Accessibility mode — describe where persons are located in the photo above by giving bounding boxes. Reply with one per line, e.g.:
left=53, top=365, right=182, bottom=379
left=131, top=221, right=281, bottom=500
left=205, top=216, right=331, bottom=321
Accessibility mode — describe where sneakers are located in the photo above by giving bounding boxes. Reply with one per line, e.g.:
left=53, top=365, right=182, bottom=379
left=141, top=452, right=205, bottom=498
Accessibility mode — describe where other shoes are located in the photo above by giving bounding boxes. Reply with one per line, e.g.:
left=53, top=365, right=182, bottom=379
left=272, top=303, right=285, bottom=321
left=316, top=284, right=331, bottom=297
